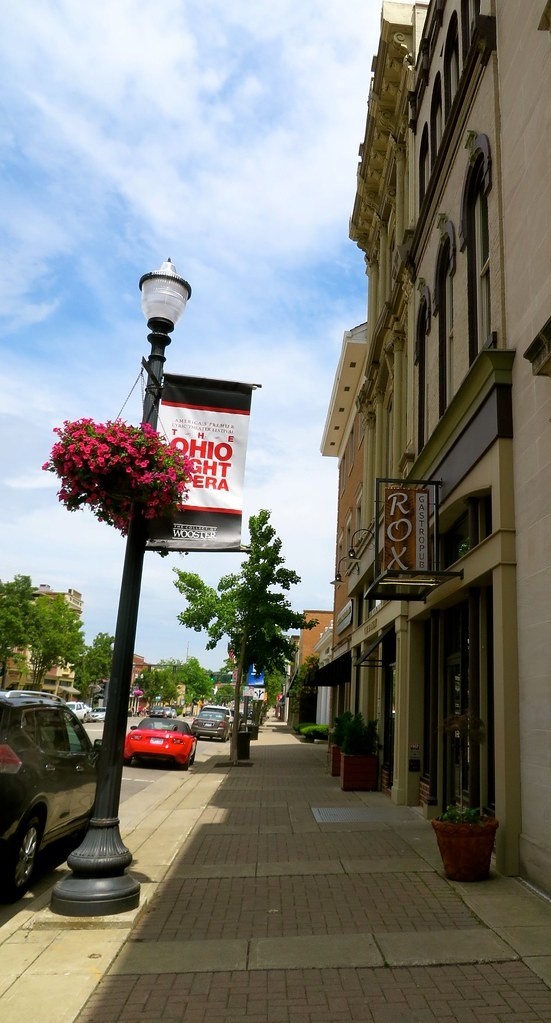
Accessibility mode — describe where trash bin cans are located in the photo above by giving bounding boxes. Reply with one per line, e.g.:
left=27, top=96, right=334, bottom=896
left=247, top=725, right=259, bottom=740
left=229, top=731, right=253, bottom=760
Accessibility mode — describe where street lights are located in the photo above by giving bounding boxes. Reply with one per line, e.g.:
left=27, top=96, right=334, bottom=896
left=47, top=258, right=193, bottom=915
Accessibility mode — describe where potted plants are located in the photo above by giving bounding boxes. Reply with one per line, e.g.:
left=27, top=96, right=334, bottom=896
left=329, top=711, right=355, bottom=777
left=339, top=712, right=381, bottom=793
left=431, top=804, right=500, bottom=882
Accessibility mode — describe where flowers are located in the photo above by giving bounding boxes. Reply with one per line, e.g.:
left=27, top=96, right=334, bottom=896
left=41, top=417, right=198, bottom=538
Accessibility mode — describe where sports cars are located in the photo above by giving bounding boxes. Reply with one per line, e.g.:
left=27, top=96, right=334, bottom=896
left=125, top=717, right=197, bottom=772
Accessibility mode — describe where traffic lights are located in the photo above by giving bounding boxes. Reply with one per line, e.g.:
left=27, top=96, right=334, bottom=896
left=231, top=675, right=232, bottom=681
left=210, top=674, right=213, bottom=681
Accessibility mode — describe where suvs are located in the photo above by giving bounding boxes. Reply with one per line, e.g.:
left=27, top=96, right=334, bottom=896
left=0, top=690, right=103, bottom=907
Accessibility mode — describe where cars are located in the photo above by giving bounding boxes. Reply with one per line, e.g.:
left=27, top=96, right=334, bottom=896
left=164, top=707, right=177, bottom=719
left=87, top=707, right=106, bottom=723
left=201, top=705, right=243, bottom=736
left=191, top=712, right=231, bottom=743
left=64, top=702, right=88, bottom=724
left=150, top=706, right=165, bottom=717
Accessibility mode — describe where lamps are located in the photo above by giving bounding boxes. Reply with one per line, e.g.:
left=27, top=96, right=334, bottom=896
left=345, top=528, right=375, bottom=562
left=330, top=557, right=360, bottom=586
